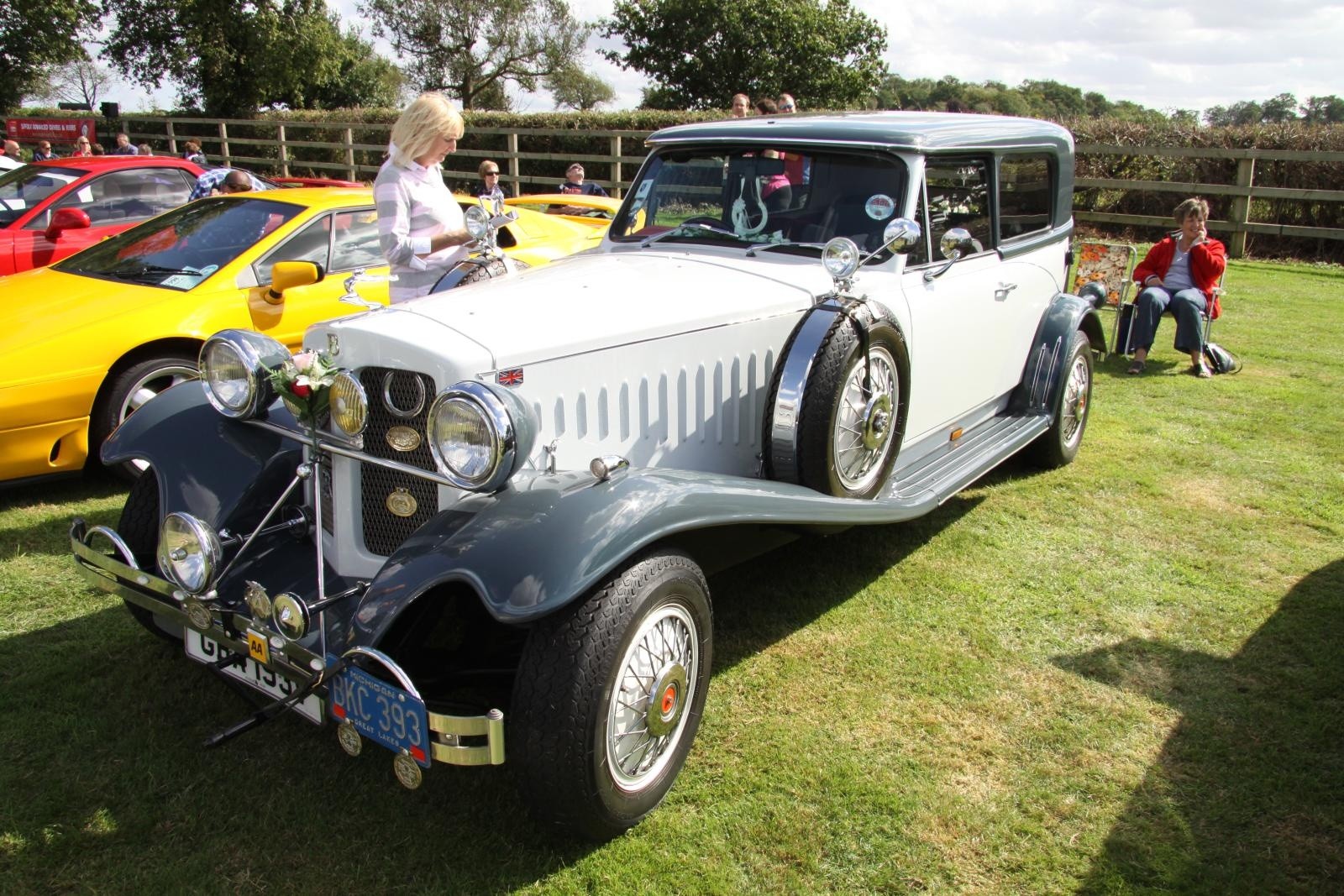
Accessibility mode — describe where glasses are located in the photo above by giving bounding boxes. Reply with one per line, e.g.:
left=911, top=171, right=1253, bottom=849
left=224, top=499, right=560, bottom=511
left=80, top=141, right=90, bottom=144
left=226, top=182, right=252, bottom=191
left=486, top=172, right=500, bottom=176
left=777, top=104, right=796, bottom=110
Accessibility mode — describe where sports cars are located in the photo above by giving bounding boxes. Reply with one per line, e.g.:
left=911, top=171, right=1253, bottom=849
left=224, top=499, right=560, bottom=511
left=0, top=153, right=370, bottom=278
left=0, top=186, right=646, bottom=494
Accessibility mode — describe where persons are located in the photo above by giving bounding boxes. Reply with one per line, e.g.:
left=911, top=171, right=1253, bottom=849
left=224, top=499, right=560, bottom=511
left=33, top=140, right=60, bottom=161
left=752, top=99, right=793, bottom=212
left=546, top=162, right=610, bottom=219
left=71, top=136, right=91, bottom=157
left=3, top=140, right=24, bottom=163
left=114, top=134, right=138, bottom=156
left=179, top=137, right=209, bottom=165
left=470, top=160, right=511, bottom=199
left=728, top=93, right=750, bottom=119
left=775, top=93, right=812, bottom=209
left=1126, top=196, right=1226, bottom=378
left=88, top=144, right=105, bottom=155
left=372, top=92, right=480, bottom=305
left=187, top=168, right=267, bottom=203
left=137, top=144, right=154, bottom=155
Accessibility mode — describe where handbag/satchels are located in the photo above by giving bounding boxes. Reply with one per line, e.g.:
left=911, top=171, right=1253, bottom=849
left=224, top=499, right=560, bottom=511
left=1203, top=342, right=1243, bottom=375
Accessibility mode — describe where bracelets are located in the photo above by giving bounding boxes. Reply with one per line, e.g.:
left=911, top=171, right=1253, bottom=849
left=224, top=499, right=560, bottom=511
left=1146, top=276, right=1158, bottom=286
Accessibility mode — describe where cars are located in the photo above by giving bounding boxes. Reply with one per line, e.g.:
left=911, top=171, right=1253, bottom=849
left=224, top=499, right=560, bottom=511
left=69, top=108, right=1109, bottom=841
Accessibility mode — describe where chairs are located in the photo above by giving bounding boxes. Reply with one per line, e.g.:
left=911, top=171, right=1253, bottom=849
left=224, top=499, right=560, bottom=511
left=1065, top=242, right=1138, bottom=364
left=259, top=213, right=390, bottom=285
left=87, top=173, right=178, bottom=227
left=1125, top=255, right=1226, bottom=365
left=782, top=181, right=1055, bottom=265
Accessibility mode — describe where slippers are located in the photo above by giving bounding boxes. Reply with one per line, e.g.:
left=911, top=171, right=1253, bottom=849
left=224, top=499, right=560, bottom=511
left=1193, top=363, right=1211, bottom=378
left=1128, top=361, right=1148, bottom=374
